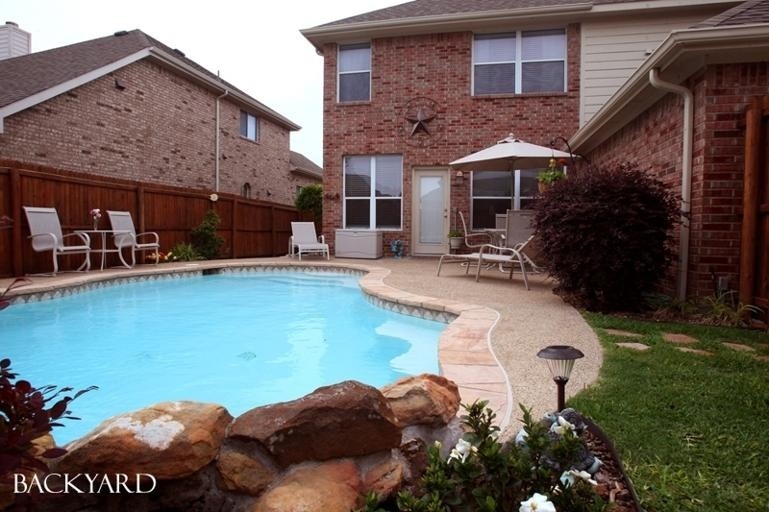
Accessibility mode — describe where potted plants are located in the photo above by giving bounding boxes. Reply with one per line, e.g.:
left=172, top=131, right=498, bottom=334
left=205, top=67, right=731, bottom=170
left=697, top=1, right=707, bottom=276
left=537, top=162, right=568, bottom=194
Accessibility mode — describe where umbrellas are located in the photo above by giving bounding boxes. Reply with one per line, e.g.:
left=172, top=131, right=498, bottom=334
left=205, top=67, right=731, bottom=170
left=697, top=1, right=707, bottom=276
left=446, top=132, right=583, bottom=209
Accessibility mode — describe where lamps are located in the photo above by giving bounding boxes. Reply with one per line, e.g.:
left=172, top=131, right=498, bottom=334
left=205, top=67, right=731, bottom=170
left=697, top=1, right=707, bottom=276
left=455, top=170, right=464, bottom=184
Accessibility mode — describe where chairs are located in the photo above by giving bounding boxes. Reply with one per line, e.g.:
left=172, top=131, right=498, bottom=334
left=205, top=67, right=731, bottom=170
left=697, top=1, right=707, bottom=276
left=22, top=205, right=160, bottom=278
left=436, top=210, right=541, bottom=291
left=287, top=221, right=330, bottom=261
